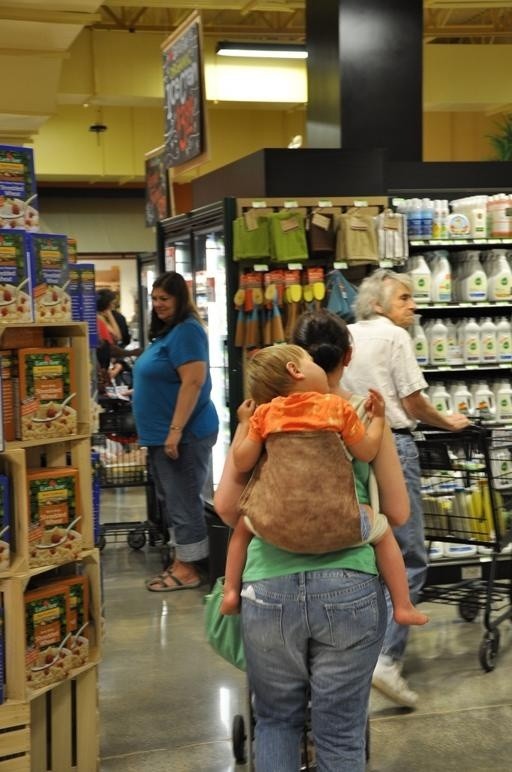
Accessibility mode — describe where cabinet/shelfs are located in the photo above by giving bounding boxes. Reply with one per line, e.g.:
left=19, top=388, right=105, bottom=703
left=1, top=320, right=101, bottom=771
left=413, top=235, right=512, bottom=586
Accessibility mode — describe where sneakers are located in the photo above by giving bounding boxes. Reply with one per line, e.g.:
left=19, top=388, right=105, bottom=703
left=371, top=652, right=418, bottom=708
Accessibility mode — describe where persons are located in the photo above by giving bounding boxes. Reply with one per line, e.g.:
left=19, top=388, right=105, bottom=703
left=218, top=341, right=428, bottom=628
left=343, top=268, right=471, bottom=710
left=211, top=310, right=411, bottom=772
left=132, top=270, right=220, bottom=592
left=94, top=287, right=132, bottom=409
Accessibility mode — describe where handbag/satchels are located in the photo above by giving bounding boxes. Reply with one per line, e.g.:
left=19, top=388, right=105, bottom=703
left=202, top=576, right=248, bottom=671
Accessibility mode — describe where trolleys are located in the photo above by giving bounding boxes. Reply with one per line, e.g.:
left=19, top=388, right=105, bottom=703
left=409, top=415, right=511, bottom=671
left=95, top=403, right=170, bottom=553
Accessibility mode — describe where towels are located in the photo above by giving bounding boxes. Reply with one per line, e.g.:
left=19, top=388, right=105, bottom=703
left=231, top=210, right=410, bottom=270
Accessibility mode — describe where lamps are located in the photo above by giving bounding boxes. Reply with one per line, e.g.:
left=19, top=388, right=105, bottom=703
left=214, top=41, right=309, bottom=59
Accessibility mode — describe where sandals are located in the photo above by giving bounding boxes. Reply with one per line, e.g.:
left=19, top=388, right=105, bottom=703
left=144, top=564, right=175, bottom=586
left=146, top=565, right=201, bottom=591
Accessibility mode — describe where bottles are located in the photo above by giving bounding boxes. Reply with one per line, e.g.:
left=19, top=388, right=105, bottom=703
left=400, top=193, right=512, bottom=240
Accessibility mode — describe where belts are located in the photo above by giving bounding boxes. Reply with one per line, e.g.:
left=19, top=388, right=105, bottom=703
left=391, top=427, right=415, bottom=437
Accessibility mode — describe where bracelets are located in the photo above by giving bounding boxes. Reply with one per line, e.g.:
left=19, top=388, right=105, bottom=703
left=167, top=423, right=184, bottom=434
